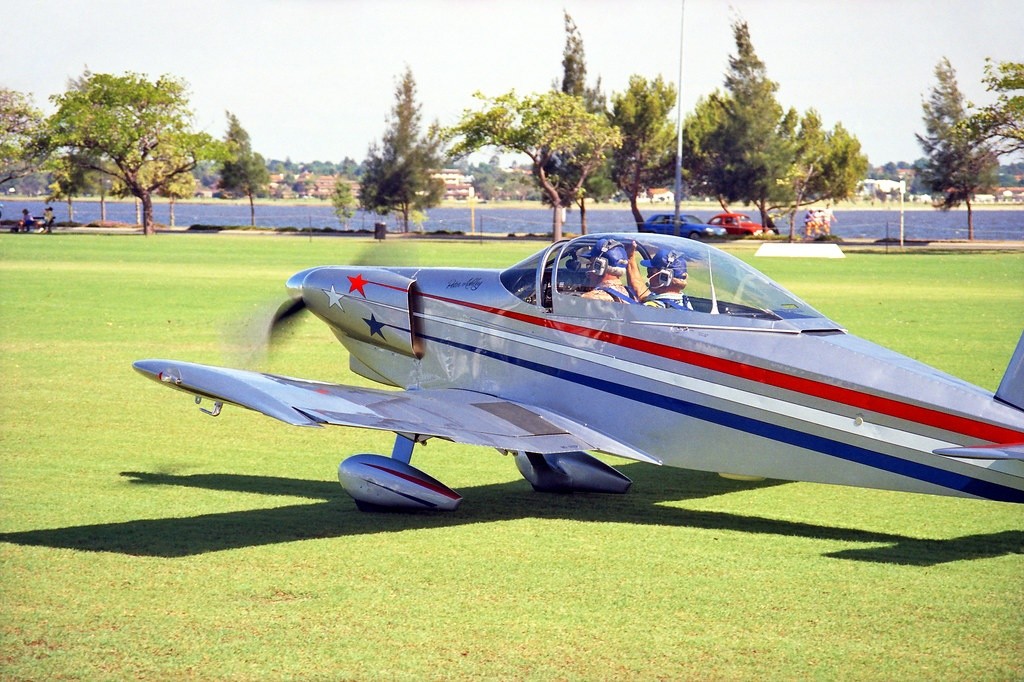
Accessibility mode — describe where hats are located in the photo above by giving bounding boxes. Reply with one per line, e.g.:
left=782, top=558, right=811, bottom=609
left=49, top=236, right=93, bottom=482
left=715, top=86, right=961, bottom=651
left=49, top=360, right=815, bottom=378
left=579, top=239, right=628, bottom=267
left=640, top=249, right=687, bottom=279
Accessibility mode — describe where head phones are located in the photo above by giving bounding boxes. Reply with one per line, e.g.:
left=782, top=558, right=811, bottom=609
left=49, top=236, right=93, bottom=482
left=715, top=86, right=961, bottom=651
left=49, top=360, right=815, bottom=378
left=592, top=239, right=625, bottom=276
left=658, top=250, right=684, bottom=286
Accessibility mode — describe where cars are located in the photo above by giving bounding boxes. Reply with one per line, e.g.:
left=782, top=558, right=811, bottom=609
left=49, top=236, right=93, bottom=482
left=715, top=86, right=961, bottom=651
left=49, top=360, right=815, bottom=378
left=640, top=212, right=726, bottom=242
left=707, top=214, right=776, bottom=238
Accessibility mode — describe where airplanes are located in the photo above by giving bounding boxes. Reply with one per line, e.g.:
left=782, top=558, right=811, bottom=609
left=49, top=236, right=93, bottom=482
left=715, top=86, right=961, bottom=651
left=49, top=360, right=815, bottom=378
left=132, top=233, right=1024, bottom=529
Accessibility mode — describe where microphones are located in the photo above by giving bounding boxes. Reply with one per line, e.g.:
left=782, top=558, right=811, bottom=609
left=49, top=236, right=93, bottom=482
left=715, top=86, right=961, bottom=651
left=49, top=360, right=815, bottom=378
left=585, top=270, right=596, bottom=278
left=646, top=282, right=665, bottom=289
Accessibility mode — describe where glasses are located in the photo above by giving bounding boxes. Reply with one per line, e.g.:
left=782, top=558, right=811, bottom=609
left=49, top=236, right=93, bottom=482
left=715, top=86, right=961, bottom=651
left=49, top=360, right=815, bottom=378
left=586, top=263, right=593, bottom=269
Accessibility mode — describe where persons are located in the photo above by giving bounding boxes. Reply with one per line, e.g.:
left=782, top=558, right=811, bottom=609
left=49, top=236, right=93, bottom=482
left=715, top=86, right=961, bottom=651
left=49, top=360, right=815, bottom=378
left=804, top=201, right=838, bottom=239
left=579, top=238, right=643, bottom=308
left=625, top=239, right=694, bottom=311
left=17, top=209, right=35, bottom=233
left=36, top=206, right=53, bottom=234
